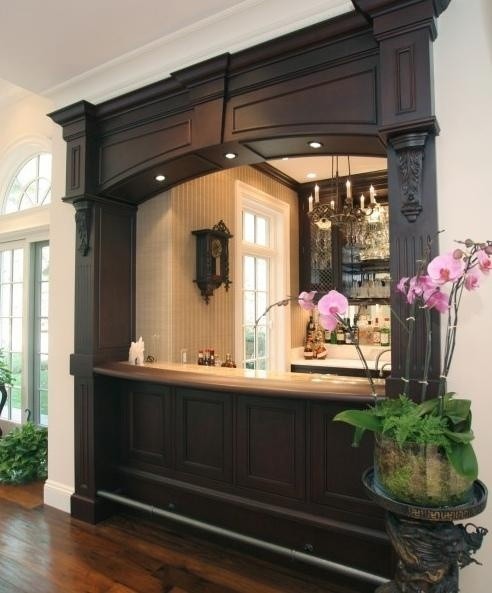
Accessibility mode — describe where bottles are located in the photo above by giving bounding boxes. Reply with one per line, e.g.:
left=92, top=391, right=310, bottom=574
left=196, top=347, right=236, bottom=368
left=302, top=315, right=393, bottom=361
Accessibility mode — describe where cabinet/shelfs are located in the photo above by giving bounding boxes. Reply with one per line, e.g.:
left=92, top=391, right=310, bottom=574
left=298, top=186, right=391, bottom=305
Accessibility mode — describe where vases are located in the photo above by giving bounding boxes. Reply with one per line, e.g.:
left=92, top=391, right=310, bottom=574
left=372, top=430, right=479, bottom=508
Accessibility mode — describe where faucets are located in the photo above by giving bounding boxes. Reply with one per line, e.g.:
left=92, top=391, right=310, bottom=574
left=374, top=348, right=391, bottom=379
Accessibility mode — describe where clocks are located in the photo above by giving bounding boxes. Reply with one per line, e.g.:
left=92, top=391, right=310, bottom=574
left=190, top=218, right=235, bottom=306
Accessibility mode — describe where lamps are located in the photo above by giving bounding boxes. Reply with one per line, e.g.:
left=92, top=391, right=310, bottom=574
left=306, top=153, right=386, bottom=247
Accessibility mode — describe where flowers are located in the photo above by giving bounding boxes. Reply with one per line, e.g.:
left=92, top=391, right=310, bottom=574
left=255, top=227, right=492, bottom=479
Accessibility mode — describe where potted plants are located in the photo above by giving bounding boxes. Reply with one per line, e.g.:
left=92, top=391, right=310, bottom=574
left=0, top=422, right=49, bottom=483
